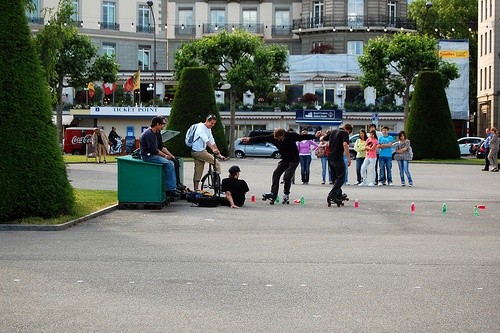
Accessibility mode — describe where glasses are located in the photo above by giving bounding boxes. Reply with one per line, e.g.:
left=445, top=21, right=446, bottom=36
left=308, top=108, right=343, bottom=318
left=162, top=123, right=166, bottom=125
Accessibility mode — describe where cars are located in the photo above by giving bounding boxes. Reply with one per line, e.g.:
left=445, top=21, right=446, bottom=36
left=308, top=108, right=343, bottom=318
left=469, top=139, right=500, bottom=159
left=227, top=136, right=283, bottom=159
left=457, top=136, right=485, bottom=156
left=348, top=132, right=397, bottom=161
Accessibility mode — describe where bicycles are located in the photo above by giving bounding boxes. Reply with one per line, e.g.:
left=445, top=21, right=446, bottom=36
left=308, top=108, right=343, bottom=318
left=200, top=152, right=226, bottom=197
left=108, top=137, right=124, bottom=156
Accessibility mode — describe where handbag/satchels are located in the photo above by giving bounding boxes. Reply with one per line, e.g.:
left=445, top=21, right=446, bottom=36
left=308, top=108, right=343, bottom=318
left=337, top=190, right=349, bottom=201
left=131, top=148, right=142, bottom=160
left=488, top=141, right=499, bottom=149
left=315, top=142, right=326, bottom=158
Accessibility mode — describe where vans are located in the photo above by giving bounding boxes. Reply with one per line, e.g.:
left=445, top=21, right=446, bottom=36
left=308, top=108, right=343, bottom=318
left=64, top=127, right=105, bottom=155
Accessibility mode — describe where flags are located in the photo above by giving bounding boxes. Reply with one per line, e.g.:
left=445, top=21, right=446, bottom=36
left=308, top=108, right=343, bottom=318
left=124, top=71, right=141, bottom=93
left=88, top=82, right=96, bottom=97
left=102, top=81, right=115, bottom=95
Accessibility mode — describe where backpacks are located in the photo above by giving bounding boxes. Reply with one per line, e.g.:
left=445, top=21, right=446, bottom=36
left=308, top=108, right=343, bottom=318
left=191, top=192, right=219, bottom=207
left=184, top=124, right=200, bottom=147
left=185, top=187, right=204, bottom=204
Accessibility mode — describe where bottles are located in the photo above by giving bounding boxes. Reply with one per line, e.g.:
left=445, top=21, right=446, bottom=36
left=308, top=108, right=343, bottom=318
left=301, top=197, right=304, bottom=205
left=474, top=206, right=478, bottom=216
left=411, top=202, right=415, bottom=211
left=442, top=203, right=446, bottom=213
left=276, top=195, right=280, bottom=203
left=355, top=199, right=358, bottom=207
left=252, top=195, right=255, bottom=202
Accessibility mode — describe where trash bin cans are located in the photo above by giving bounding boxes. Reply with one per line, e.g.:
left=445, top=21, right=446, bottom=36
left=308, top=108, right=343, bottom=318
left=161, top=131, right=184, bottom=208
left=116, top=155, right=170, bottom=210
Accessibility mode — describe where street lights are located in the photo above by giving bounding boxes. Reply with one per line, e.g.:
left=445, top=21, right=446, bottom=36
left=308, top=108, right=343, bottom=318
left=147, top=0, right=157, bottom=107
left=420, top=1, right=433, bottom=36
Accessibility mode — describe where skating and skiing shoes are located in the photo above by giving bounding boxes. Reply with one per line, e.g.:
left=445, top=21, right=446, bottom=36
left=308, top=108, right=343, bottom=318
left=282, top=195, right=290, bottom=205
left=327, top=193, right=344, bottom=207
left=262, top=192, right=278, bottom=205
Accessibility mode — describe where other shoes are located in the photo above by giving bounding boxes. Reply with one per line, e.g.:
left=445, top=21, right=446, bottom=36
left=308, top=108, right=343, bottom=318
left=166, top=190, right=180, bottom=197
left=490, top=166, right=497, bottom=172
left=358, top=182, right=367, bottom=186
left=383, top=181, right=386, bottom=185
left=280, top=180, right=295, bottom=184
left=330, top=180, right=334, bottom=184
left=322, top=181, right=325, bottom=184
left=345, top=182, right=350, bottom=185
left=388, top=183, right=393, bottom=185
left=302, top=181, right=308, bottom=184
left=354, top=182, right=361, bottom=185
left=402, top=184, right=405, bottom=186
left=408, top=184, right=412, bottom=187
left=368, top=182, right=374, bottom=186
left=482, top=168, right=489, bottom=171
left=176, top=183, right=186, bottom=190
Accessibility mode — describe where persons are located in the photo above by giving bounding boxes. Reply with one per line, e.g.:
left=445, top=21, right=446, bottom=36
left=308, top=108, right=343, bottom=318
left=320, top=124, right=353, bottom=207
left=318, top=129, right=351, bottom=186
left=297, top=131, right=318, bottom=184
left=241, top=127, right=321, bottom=205
left=192, top=114, right=225, bottom=193
left=395, top=131, right=413, bottom=186
left=108, top=126, right=122, bottom=151
left=187, top=165, right=249, bottom=209
left=377, top=126, right=394, bottom=186
left=354, top=124, right=382, bottom=186
left=92, top=127, right=109, bottom=163
left=140, top=116, right=186, bottom=197
left=481, top=127, right=499, bottom=172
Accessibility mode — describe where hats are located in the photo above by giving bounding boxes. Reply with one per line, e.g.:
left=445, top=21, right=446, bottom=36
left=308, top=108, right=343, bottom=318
left=93, top=127, right=99, bottom=132
left=229, top=166, right=241, bottom=174
